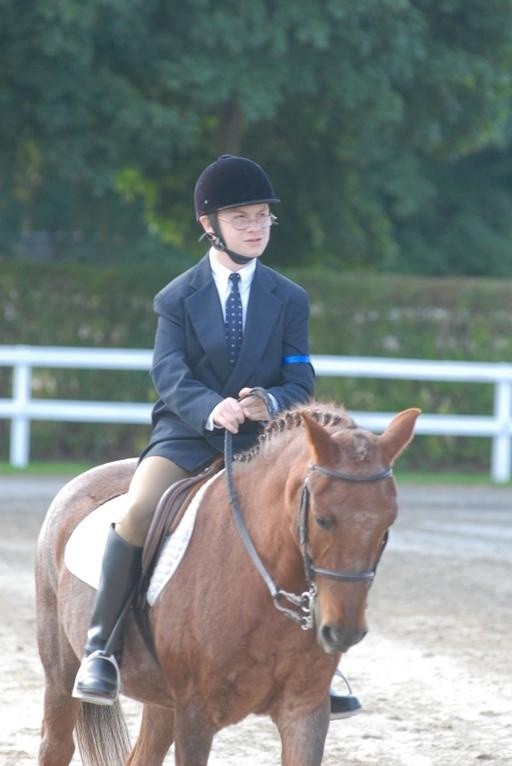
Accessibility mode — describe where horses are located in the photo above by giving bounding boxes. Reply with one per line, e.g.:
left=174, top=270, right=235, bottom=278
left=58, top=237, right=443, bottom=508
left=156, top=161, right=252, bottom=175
left=34, top=397, right=421, bottom=766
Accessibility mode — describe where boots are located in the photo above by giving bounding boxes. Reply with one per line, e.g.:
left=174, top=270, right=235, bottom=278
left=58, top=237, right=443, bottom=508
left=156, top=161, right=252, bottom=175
left=76, top=523, right=144, bottom=699
left=329, top=691, right=360, bottom=713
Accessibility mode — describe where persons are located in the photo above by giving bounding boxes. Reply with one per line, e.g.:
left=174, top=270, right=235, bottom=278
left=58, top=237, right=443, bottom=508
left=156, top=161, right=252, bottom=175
left=72, top=155, right=364, bottom=716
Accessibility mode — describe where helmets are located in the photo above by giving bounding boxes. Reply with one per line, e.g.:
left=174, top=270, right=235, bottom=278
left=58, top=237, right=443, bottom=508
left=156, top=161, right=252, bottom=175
left=194, top=154, right=280, bottom=224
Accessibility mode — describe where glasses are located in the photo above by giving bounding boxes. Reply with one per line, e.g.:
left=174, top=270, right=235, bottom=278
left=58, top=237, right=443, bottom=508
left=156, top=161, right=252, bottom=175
left=216, top=212, right=278, bottom=230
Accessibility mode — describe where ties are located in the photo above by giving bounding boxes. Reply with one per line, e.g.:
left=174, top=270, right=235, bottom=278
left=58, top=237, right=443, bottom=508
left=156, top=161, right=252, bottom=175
left=225, top=273, right=243, bottom=366
